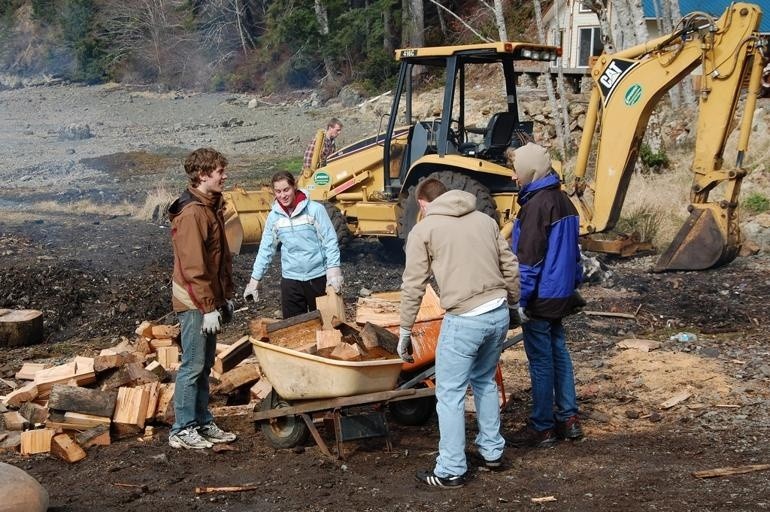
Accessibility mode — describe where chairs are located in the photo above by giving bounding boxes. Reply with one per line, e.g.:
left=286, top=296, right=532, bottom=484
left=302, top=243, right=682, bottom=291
left=450, top=113, right=517, bottom=166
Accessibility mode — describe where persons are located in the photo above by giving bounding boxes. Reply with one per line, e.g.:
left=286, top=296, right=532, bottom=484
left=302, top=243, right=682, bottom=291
left=304, top=118, right=343, bottom=169
left=503, top=140, right=585, bottom=447
left=243, top=169, right=344, bottom=320
left=395, top=176, right=523, bottom=489
left=167, top=148, right=240, bottom=450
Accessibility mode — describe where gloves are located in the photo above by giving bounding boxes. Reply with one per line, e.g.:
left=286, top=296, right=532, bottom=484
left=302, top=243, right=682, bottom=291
left=509, top=305, right=530, bottom=329
left=396, top=328, right=415, bottom=363
left=243, top=278, right=262, bottom=305
left=324, top=266, right=344, bottom=294
left=203, top=309, right=222, bottom=335
left=221, top=299, right=234, bottom=324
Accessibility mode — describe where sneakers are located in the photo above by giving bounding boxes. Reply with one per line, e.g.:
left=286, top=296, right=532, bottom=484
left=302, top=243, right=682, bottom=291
left=199, top=421, right=237, bottom=443
left=504, top=424, right=556, bottom=449
left=168, top=426, right=214, bottom=449
left=415, top=470, right=464, bottom=488
left=554, top=414, right=584, bottom=439
left=484, top=453, right=504, bottom=467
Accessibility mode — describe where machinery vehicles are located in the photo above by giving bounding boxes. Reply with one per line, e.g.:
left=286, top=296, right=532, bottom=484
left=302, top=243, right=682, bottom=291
left=219, top=0, right=769, bottom=275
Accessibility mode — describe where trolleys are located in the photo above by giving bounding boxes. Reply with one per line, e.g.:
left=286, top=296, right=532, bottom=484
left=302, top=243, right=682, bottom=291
left=239, top=336, right=439, bottom=446
